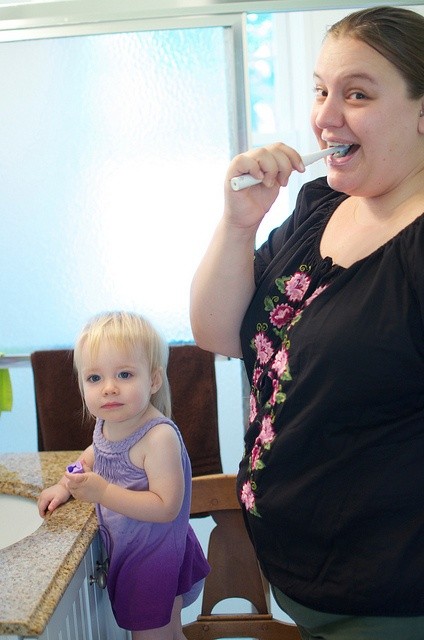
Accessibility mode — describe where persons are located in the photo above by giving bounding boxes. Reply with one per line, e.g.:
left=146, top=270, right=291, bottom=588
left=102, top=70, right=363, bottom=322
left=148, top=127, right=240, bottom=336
left=37, top=311, right=210, bottom=640
left=189, top=7, right=424, bottom=639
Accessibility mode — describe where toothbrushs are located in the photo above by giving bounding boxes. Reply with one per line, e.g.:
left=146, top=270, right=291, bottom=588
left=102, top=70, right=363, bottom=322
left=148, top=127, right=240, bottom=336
left=231, top=144, right=352, bottom=191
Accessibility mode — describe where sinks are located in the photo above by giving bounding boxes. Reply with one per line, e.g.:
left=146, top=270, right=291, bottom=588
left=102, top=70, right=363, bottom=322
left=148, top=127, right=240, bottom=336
left=1, top=480, right=47, bottom=550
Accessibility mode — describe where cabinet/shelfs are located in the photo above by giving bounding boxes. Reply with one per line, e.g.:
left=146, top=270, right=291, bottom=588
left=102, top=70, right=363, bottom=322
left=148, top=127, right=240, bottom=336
left=0, top=530, right=132, bottom=640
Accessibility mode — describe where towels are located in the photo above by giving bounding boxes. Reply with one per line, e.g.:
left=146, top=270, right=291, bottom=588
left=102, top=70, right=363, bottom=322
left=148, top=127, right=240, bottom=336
left=30, top=343, right=224, bottom=518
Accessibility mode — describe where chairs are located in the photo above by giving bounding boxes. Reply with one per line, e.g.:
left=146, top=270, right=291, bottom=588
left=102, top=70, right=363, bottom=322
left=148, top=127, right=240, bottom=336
left=181, top=473, right=303, bottom=640
